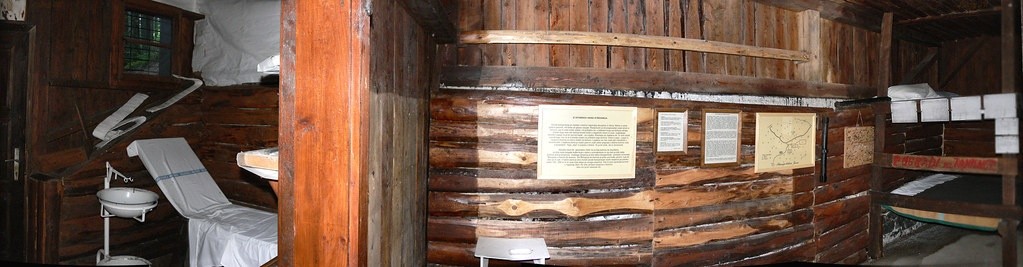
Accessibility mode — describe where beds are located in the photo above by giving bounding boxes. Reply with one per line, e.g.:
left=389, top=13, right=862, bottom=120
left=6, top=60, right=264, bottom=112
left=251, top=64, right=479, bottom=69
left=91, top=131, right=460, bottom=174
left=127, top=137, right=280, bottom=267
left=870, top=0, right=1023, bottom=267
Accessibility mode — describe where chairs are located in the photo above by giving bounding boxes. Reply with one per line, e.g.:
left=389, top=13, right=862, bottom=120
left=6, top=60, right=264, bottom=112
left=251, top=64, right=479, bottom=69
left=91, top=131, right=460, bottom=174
left=474, top=236, right=550, bottom=267
